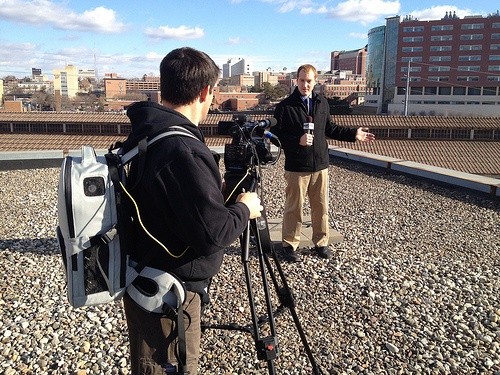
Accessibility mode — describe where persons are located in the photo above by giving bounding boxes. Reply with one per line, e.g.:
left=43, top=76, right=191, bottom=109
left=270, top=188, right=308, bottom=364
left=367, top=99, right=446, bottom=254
left=269, top=64, right=376, bottom=263
left=106, top=46, right=265, bottom=375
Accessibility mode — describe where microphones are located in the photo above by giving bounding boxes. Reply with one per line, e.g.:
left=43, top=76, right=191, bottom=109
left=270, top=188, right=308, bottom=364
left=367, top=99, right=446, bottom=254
left=302, top=115, right=314, bottom=134
left=256, top=118, right=277, bottom=129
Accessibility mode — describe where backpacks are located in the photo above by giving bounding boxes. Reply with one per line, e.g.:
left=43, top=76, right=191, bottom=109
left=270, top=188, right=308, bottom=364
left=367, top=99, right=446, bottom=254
left=56, top=125, right=215, bottom=309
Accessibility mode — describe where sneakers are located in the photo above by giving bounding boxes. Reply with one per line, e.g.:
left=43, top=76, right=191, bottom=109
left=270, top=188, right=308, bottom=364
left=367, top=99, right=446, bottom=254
left=314, top=245, right=332, bottom=259
left=283, top=246, right=298, bottom=261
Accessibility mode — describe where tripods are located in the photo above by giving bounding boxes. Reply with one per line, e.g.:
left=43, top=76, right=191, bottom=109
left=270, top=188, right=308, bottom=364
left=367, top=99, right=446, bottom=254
left=200, top=214, right=321, bottom=375
